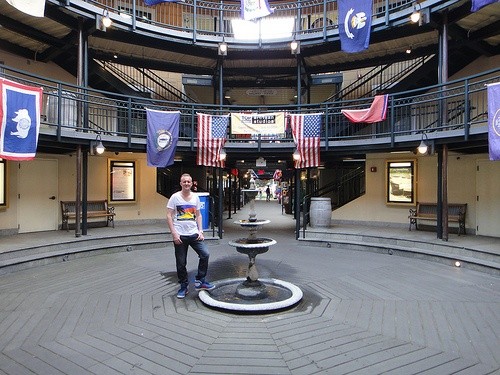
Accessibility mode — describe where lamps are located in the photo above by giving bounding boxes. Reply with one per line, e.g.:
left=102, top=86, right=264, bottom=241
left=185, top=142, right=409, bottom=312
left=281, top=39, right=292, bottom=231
left=220, top=35, right=227, bottom=55
left=91, top=135, right=106, bottom=156
left=100, top=8, right=112, bottom=30
left=417, top=133, right=429, bottom=153
left=291, top=34, right=298, bottom=53
left=292, top=150, right=300, bottom=160
left=220, top=149, right=227, bottom=161
left=411, top=2, right=426, bottom=23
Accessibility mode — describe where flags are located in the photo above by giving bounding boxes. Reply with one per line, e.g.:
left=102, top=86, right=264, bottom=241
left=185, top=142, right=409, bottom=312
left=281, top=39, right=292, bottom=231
left=240, top=0, right=273, bottom=20
left=487, top=83, right=500, bottom=160
left=337, top=0, right=373, bottom=53
left=289, top=114, right=321, bottom=168
left=341, top=96, right=390, bottom=123
left=0, top=76, right=42, bottom=161
left=146, top=109, right=180, bottom=168
left=196, top=114, right=228, bottom=167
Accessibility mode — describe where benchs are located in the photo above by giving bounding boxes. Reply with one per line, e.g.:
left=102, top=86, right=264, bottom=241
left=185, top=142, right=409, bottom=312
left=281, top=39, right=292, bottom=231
left=60, top=200, right=116, bottom=232
left=408, top=202, right=467, bottom=236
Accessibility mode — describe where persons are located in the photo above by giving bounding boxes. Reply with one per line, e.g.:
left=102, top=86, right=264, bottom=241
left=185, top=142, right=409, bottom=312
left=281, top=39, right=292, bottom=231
left=266, top=185, right=271, bottom=202
left=168, top=173, right=215, bottom=298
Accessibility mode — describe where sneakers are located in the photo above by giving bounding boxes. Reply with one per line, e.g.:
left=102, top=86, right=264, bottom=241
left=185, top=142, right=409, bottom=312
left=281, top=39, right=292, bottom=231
left=195, top=279, right=215, bottom=290
left=177, top=288, right=189, bottom=298
